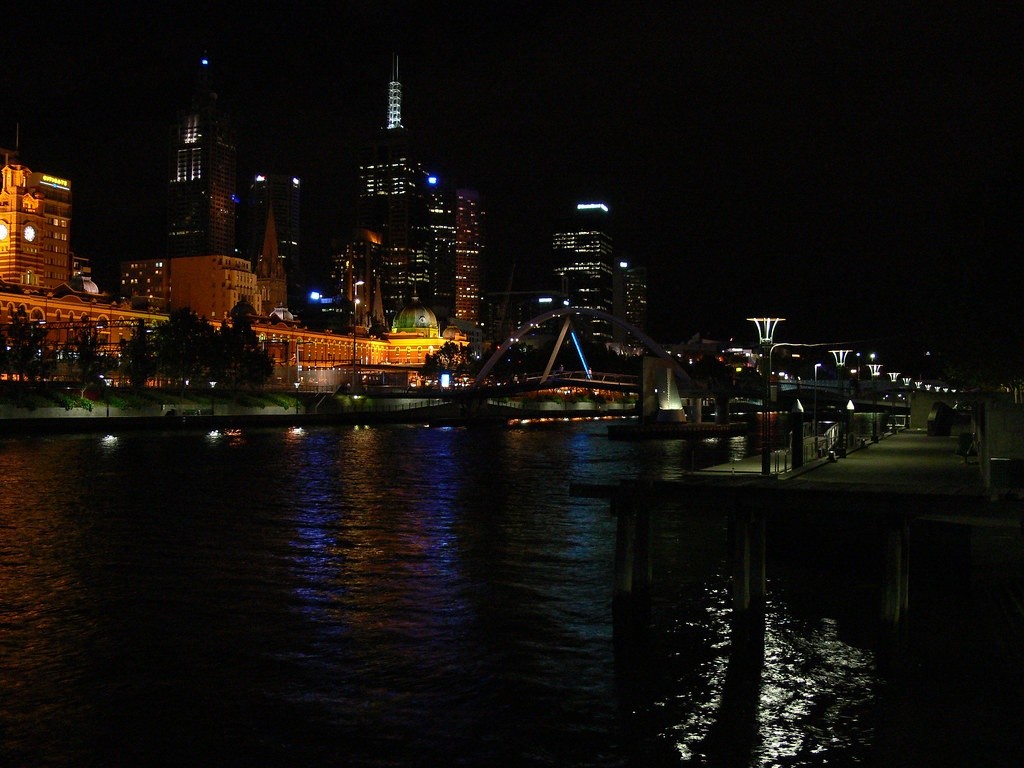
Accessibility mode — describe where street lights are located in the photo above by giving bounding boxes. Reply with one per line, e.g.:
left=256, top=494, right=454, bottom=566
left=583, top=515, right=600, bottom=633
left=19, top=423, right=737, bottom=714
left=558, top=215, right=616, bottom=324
left=827, top=349, right=854, bottom=458
left=866, top=364, right=884, bottom=443
left=746, top=317, right=787, bottom=476
left=353, top=281, right=365, bottom=385
left=888, top=372, right=902, bottom=435
left=900, top=376, right=913, bottom=429
